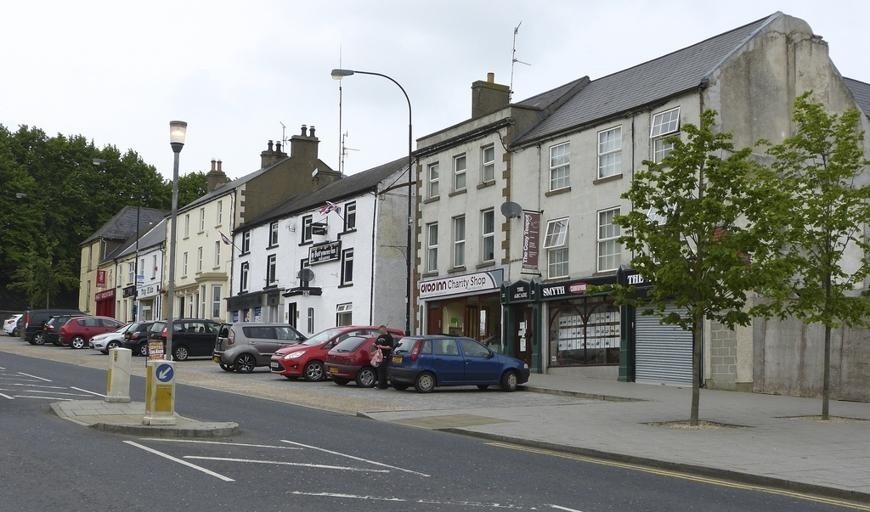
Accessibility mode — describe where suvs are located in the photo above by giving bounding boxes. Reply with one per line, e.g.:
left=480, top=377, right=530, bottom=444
left=124, top=320, right=155, bottom=357
left=150, top=315, right=227, bottom=361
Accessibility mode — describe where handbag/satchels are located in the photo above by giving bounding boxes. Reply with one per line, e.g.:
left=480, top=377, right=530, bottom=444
left=370, top=345, right=383, bottom=368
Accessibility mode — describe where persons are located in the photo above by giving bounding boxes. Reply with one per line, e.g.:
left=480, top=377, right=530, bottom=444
left=372, top=325, right=393, bottom=389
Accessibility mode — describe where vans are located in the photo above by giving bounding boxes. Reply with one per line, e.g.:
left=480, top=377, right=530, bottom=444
left=16, top=306, right=90, bottom=345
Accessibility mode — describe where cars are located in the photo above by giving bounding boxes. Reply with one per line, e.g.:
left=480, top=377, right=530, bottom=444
left=88, top=322, right=134, bottom=355
left=2, top=312, right=25, bottom=337
left=43, top=314, right=127, bottom=346
left=270, top=324, right=407, bottom=380
left=390, top=334, right=533, bottom=393
left=60, top=316, right=126, bottom=348
left=215, top=322, right=312, bottom=376
left=327, top=333, right=396, bottom=389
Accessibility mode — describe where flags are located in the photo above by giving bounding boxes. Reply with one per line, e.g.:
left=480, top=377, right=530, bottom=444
left=318, top=201, right=342, bottom=216
left=222, top=233, right=233, bottom=246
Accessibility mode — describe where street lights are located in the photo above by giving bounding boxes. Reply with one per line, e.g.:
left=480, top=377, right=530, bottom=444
left=330, top=65, right=418, bottom=337
left=91, top=157, right=141, bottom=322
left=159, top=116, right=190, bottom=357
left=15, top=191, right=34, bottom=310
